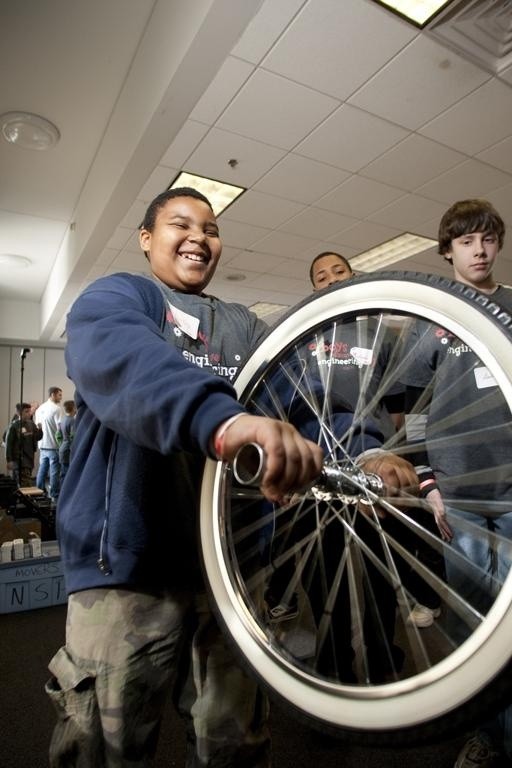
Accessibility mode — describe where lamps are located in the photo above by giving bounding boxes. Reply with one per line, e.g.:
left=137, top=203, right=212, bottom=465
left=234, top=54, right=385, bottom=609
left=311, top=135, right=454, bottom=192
left=0, top=110, right=61, bottom=152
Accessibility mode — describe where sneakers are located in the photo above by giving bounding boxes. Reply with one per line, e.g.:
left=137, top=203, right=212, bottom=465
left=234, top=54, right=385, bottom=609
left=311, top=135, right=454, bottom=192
left=262, top=604, right=300, bottom=625
left=406, top=605, right=441, bottom=628
left=454, top=730, right=499, bottom=766
